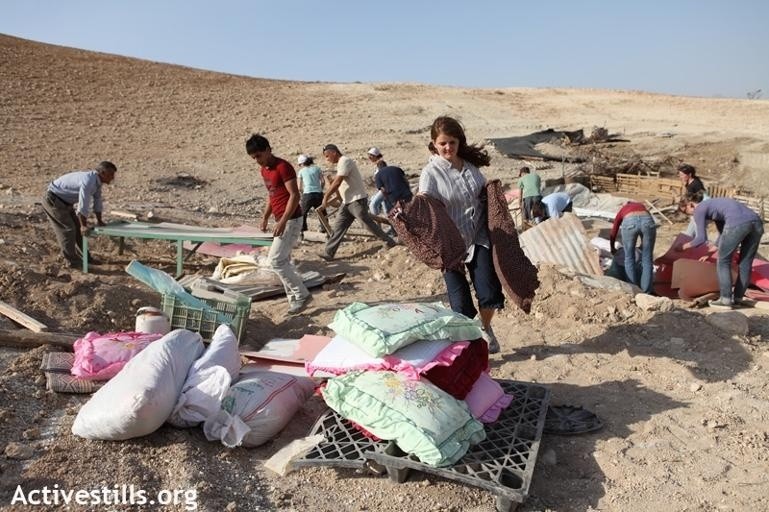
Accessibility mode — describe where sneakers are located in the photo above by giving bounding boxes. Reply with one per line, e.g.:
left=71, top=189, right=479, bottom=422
left=708, top=297, right=743, bottom=311
left=480, top=323, right=503, bottom=354
left=316, top=251, right=335, bottom=263
left=287, top=293, right=314, bottom=316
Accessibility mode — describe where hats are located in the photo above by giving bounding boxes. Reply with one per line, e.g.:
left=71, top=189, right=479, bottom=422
left=367, top=146, right=382, bottom=157
left=322, top=143, right=344, bottom=156
left=296, top=153, right=309, bottom=166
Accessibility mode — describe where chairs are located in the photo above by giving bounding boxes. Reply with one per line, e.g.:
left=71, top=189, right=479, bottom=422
left=314, top=174, right=343, bottom=233
left=506, top=188, right=525, bottom=228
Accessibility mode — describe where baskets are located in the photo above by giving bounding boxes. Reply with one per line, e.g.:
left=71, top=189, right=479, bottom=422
left=162, top=292, right=252, bottom=348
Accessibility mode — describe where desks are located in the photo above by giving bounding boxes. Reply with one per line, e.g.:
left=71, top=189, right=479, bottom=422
left=80, top=220, right=276, bottom=278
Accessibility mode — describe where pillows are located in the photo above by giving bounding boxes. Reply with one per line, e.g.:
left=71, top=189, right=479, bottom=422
left=71, top=331, right=164, bottom=379
left=308, top=299, right=512, bottom=467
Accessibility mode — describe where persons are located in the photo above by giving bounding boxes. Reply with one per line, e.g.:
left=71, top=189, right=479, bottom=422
left=679, top=165, right=710, bottom=237
left=393, top=116, right=511, bottom=353
left=673, top=191, right=764, bottom=310
left=516, top=167, right=543, bottom=223
left=296, top=154, right=330, bottom=234
left=369, top=147, right=397, bottom=234
left=533, top=193, right=572, bottom=220
left=609, top=201, right=657, bottom=294
left=246, top=132, right=313, bottom=315
left=375, top=161, right=413, bottom=219
left=42, top=160, right=118, bottom=271
left=315, top=144, right=398, bottom=261
